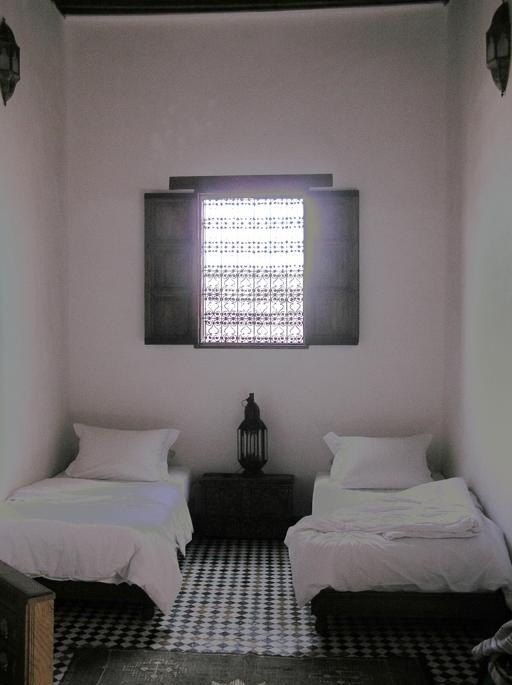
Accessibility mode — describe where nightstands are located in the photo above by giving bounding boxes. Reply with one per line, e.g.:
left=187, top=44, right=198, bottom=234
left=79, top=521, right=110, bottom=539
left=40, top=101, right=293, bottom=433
left=198, top=473, right=293, bottom=540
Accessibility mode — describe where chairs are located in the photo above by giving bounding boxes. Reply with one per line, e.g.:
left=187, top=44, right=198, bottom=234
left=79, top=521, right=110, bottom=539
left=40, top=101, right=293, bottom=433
left=0, top=559, right=55, bottom=685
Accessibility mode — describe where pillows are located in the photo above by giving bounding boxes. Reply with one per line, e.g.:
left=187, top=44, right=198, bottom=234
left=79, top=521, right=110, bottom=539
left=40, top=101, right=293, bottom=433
left=63, top=422, right=181, bottom=481
left=322, top=432, right=435, bottom=489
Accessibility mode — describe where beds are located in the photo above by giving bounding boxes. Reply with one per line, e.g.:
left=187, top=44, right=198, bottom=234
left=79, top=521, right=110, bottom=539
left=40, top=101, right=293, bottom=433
left=0, top=464, right=194, bottom=616
left=284, top=471, right=512, bottom=635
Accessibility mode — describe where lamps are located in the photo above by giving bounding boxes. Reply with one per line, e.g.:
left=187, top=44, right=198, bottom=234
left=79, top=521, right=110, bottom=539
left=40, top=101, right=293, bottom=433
left=237, top=393, right=268, bottom=478
left=0, top=16, right=20, bottom=107
left=486, top=0, right=512, bottom=98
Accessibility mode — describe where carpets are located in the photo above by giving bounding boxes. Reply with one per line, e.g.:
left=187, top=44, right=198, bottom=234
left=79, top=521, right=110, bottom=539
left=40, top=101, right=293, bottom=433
left=59, top=646, right=434, bottom=685
